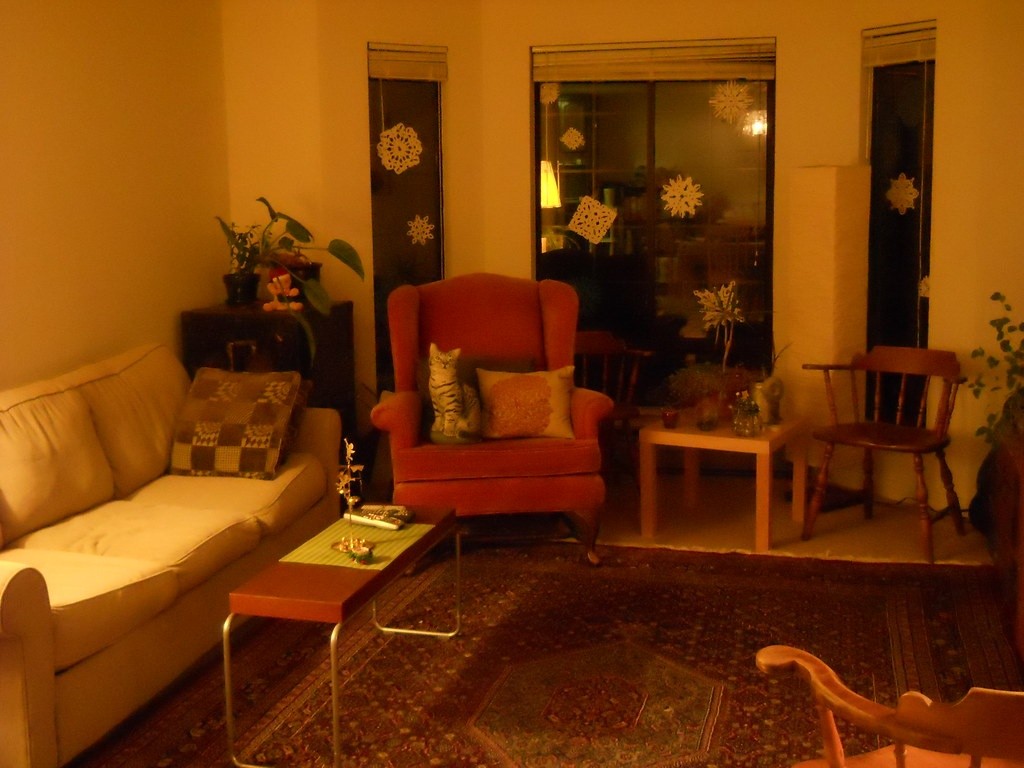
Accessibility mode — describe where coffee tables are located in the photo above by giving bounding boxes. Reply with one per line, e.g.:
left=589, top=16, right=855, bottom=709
left=222, top=503, right=461, bottom=768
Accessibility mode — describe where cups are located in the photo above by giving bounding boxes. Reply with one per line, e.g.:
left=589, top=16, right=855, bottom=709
left=662, top=409, right=680, bottom=428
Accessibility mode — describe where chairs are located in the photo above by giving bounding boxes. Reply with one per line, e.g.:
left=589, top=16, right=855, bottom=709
left=756, top=645, right=1024, bottom=768
left=800, top=345, right=967, bottom=562
left=574, top=331, right=653, bottom=491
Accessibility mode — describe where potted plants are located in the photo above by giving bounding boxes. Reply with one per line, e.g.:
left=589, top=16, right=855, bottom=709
left=214, top=196, right=364, bottom=364
left=660, top=280, right=794, bottom=438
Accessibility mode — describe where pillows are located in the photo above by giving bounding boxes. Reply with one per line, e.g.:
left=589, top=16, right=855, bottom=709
left=169, top=366, right=312, bottom=481
left=475, top=367, right=576, bottom=440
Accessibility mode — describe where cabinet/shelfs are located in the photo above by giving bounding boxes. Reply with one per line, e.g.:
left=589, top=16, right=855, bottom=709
left=181, top=298, right=356, bottom=463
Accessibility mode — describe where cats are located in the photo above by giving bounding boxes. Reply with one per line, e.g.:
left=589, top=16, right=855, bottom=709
left=428, top=344, right=481, bottom=437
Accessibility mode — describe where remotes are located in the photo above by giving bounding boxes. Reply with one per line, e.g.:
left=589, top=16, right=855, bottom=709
left=360, top=510, right=416, bottom=523
left=361, top=504, right=406, bottom=511
left=345, top=510, right=404, bottom=530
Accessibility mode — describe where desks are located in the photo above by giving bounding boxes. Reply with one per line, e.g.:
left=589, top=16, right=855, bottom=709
left=640, top=410, right=809, bottom=552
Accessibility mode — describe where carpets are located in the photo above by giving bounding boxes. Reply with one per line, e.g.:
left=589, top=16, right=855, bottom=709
left=61, top=535, right=1024, bottom=768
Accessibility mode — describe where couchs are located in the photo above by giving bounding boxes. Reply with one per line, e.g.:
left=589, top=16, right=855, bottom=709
left=0, top=343, right=342, bottom=768
left=369, top=271, right=616, bottom=576
